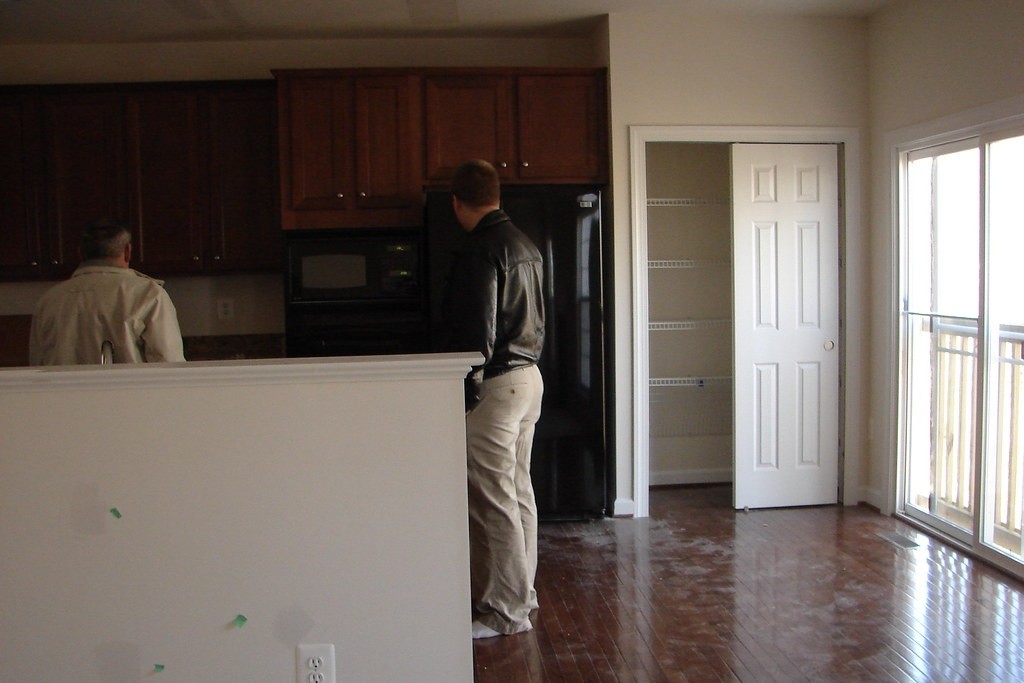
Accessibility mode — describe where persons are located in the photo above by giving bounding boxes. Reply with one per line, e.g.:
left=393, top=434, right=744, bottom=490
left=28, top=224, right=189, bottom=368
left=450, top=160, right=546, bottom=640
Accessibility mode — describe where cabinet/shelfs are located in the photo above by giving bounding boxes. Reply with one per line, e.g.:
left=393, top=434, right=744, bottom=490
left=0, top=67, right=608, bottom=282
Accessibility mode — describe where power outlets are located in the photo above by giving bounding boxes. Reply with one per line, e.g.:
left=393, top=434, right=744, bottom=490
left=216, top=299, right=234, bottom=320
left=296, top=644, right=335, bottom=683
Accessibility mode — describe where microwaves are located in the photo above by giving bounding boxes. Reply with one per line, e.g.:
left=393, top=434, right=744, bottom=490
left=284, top=231, right=421, bottom=299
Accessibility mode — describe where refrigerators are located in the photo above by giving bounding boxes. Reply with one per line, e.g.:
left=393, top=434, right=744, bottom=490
left=423, top=177, right=610, bottom=523
